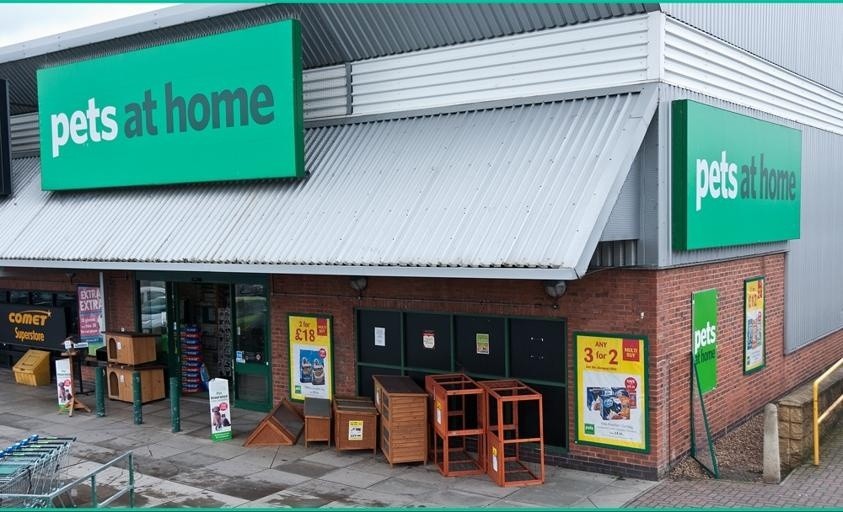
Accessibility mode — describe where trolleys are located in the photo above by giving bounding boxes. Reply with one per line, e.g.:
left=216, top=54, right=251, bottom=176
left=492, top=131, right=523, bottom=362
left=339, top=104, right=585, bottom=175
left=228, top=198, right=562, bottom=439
left=0, top=434, right=76, bottom=507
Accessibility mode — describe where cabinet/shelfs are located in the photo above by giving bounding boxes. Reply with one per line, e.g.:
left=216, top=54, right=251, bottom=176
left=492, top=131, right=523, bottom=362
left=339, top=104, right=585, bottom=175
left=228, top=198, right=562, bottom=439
left=477, top=378, right=545, bottom=487
left=372, top=375, right=430, bottom=467
left=334, top=393, right=379, bottom=459
left=425, top=373, right=487, bottom=477
left=303, top=397, right=332, bottom=449
